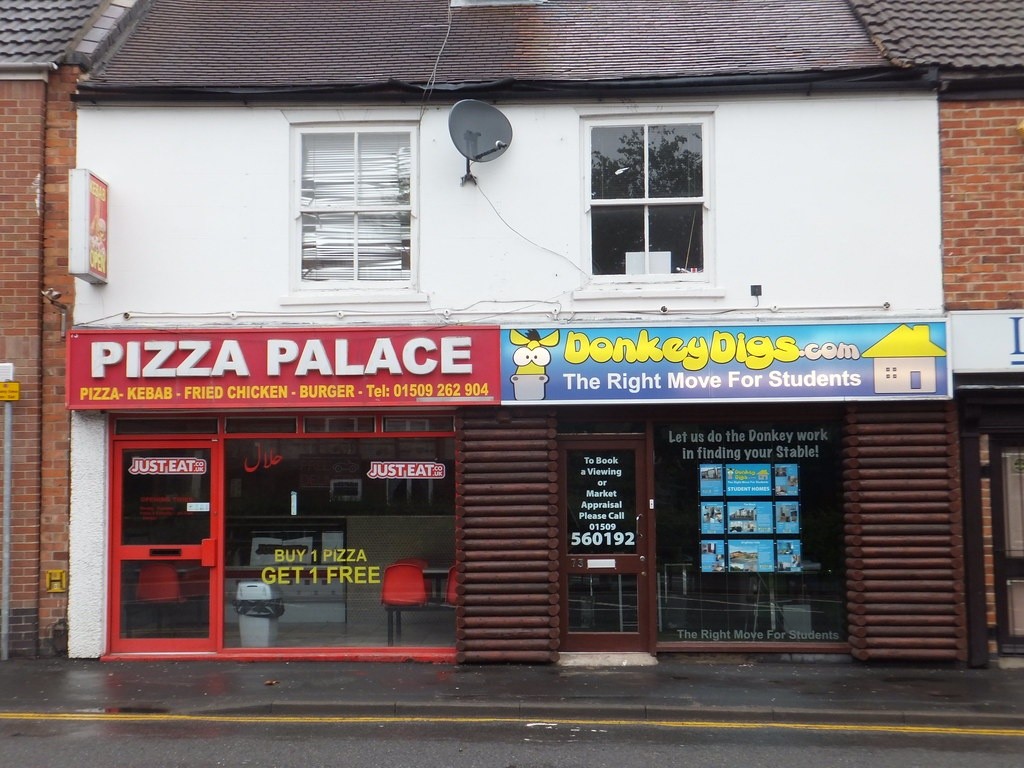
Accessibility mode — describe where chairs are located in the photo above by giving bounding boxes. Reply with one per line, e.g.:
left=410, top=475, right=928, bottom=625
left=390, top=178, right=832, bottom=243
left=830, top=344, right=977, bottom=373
left=137, top=562, right=210, bottom=637
left=381, top=557, right=459, bottom=647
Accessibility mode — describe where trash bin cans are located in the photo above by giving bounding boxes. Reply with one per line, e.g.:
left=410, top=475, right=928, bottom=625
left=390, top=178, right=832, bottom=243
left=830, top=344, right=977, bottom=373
left=235, top=580, right=284, bottom=648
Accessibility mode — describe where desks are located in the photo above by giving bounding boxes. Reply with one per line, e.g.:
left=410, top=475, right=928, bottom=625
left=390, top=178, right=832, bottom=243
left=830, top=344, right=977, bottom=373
left=132, top=568, right=209, bottom=634
left=385, top=567, right=459, bottom=639
